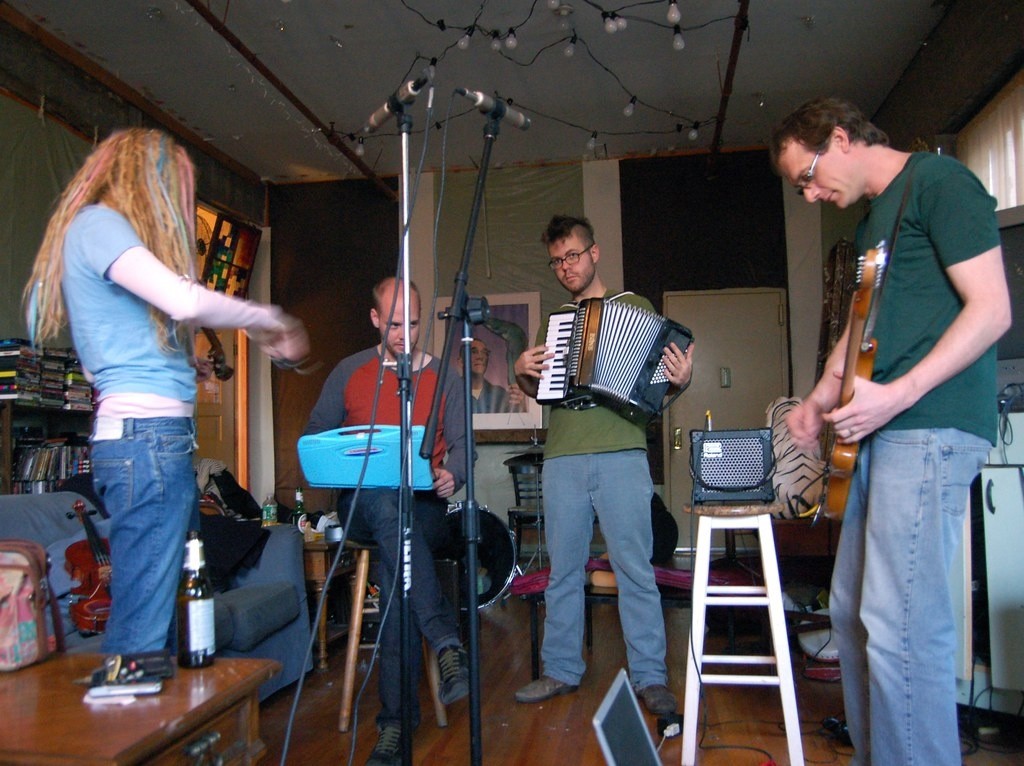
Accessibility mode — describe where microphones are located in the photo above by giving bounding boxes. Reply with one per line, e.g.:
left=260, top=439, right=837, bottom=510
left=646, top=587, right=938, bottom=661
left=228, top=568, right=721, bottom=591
left=456, top=87, right=531, bottom=130
left=362, top=77, right=429, bottom=134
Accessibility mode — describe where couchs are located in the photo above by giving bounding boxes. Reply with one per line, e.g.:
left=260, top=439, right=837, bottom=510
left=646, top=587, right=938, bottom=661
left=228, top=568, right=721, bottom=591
left=0, top=492, right=314, bottom=706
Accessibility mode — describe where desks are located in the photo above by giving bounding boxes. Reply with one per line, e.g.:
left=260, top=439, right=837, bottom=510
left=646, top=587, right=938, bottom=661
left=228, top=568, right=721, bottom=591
left=303, top=537, right=361, bottom=669
left=769, top=518, right=842, bottom=638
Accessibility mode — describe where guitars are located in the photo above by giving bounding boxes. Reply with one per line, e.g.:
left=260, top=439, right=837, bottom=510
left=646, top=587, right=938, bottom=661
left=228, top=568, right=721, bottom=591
left=820, top=239, right=891, bottom=522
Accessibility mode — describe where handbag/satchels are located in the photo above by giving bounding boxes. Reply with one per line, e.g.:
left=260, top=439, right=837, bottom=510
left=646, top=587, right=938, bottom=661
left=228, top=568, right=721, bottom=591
left=0, top=540, right=59, bottom=671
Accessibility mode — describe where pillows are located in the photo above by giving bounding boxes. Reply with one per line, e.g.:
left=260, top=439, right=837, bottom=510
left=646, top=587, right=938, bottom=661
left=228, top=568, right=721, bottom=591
left=47, top=517, right=111, bottom=597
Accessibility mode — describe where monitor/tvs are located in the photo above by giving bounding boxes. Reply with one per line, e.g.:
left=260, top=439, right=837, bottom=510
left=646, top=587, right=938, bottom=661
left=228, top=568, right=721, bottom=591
left=992, top=205, right=1024, bottom=397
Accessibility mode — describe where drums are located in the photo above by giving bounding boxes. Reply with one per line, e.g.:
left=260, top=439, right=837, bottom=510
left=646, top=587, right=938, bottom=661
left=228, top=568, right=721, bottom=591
left=442, top=499, right=519, bottom=613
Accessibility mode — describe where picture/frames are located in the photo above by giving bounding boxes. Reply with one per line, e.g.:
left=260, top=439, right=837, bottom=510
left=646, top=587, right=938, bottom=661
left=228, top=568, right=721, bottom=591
left=432, top=291, right=544, bottom=430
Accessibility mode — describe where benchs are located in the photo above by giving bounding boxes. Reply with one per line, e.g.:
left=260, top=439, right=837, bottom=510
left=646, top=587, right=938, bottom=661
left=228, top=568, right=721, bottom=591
left=518, top=583, right=737, bottom=689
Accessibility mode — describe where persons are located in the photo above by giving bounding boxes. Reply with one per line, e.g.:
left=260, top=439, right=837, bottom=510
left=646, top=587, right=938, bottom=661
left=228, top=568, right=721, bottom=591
left=20, top=125, right=309, bottom=653
left=512, top=213, right=695, bottom=715
left=302, top=275, right=477, bottom=766
left=457, top=337, right=527, bottom=415
left=769, top=95, right=1013, bottom=766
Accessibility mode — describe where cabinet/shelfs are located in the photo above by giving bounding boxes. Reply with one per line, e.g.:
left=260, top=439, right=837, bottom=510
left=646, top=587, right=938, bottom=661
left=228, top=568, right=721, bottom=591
left=954, top=413, right=1024, bottom=744
left=0, top=656, right=284, bottom=766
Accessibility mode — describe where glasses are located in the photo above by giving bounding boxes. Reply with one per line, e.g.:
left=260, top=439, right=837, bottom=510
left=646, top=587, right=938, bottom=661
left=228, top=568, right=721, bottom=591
left=548, top=243, right=594, bottom=270
left=794, top=151, right=821, bottom=195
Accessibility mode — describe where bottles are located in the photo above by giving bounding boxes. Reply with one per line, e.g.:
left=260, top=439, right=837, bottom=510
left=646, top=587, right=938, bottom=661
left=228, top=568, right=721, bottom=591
left=260, top=493, right=277, bottom=526
left=177, top=531, right=216, bottom=669
left=288, top=485, right=306, bottom=535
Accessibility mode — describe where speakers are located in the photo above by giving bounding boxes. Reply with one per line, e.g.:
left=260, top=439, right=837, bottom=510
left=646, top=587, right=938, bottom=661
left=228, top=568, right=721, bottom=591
left=690, top=429, right=774, bottom=505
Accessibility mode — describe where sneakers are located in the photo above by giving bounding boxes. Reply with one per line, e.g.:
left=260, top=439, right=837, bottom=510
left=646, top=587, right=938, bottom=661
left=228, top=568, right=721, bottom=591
left=365, top=721, right=415, bottom=766
left=636, top=683, right=680, bottom=716
left=434, top=645, right=470, bottom=705
left=514, top=674, right=580, bottom=704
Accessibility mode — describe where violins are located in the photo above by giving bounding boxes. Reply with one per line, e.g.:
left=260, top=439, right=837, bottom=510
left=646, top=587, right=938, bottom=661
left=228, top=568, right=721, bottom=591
left=61, top=498, right=115, bottom=635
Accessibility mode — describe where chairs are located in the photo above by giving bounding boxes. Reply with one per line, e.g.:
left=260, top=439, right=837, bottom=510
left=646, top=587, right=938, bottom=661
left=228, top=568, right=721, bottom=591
left=503, top=451, right=544, bottom=563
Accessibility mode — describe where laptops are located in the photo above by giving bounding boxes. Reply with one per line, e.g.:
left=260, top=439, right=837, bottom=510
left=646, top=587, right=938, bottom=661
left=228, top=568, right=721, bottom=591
left=593, top=667, right=663, bottom=766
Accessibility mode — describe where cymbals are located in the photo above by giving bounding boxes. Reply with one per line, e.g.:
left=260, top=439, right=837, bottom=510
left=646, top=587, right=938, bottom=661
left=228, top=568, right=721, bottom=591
left=504, top=443, right=549, bottom=457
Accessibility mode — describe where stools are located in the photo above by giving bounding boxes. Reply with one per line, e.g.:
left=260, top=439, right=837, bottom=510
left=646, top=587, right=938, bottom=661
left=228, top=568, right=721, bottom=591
left=339, top=539, right=448, bottom=733
left=681, top=502, right=805, bottom=766
